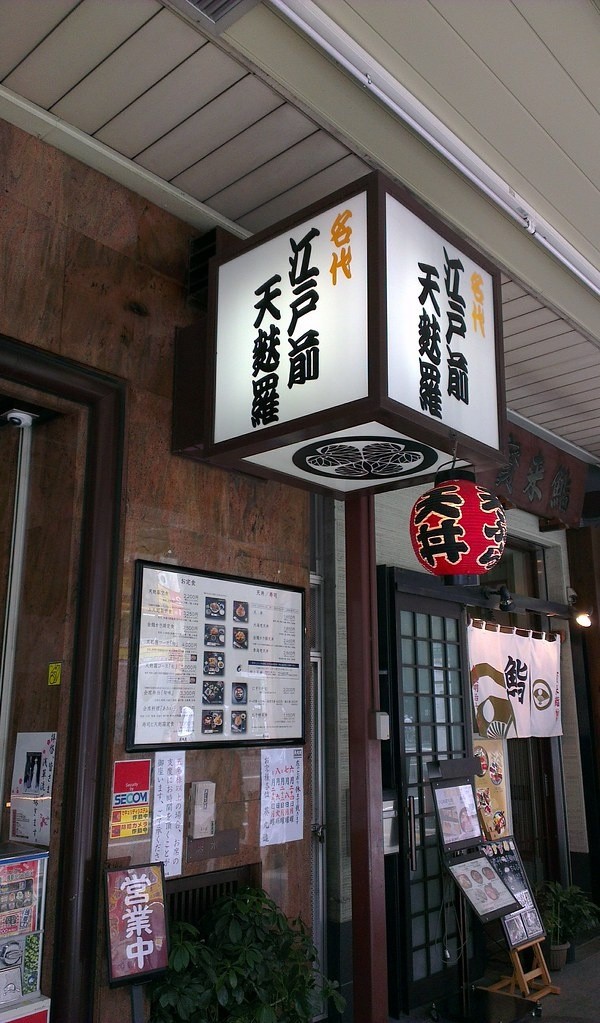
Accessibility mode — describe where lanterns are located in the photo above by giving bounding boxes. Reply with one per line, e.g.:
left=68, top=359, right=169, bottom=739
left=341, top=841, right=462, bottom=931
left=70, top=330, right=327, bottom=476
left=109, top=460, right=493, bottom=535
left=411, top=482, right=508, bottom=586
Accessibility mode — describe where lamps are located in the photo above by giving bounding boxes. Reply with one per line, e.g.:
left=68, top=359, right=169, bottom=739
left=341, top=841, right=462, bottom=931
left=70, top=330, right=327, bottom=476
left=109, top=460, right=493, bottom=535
left=168, top=168, right=511, bottom=500
left=484, top=586, right=516, bottom=611
left=268, top=1, right=599, bottom=299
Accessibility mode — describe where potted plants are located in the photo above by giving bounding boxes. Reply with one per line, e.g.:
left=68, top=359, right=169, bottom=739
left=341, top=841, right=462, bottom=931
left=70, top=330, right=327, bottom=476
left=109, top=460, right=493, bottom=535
left=532, top=880, right=600, bottom=970
